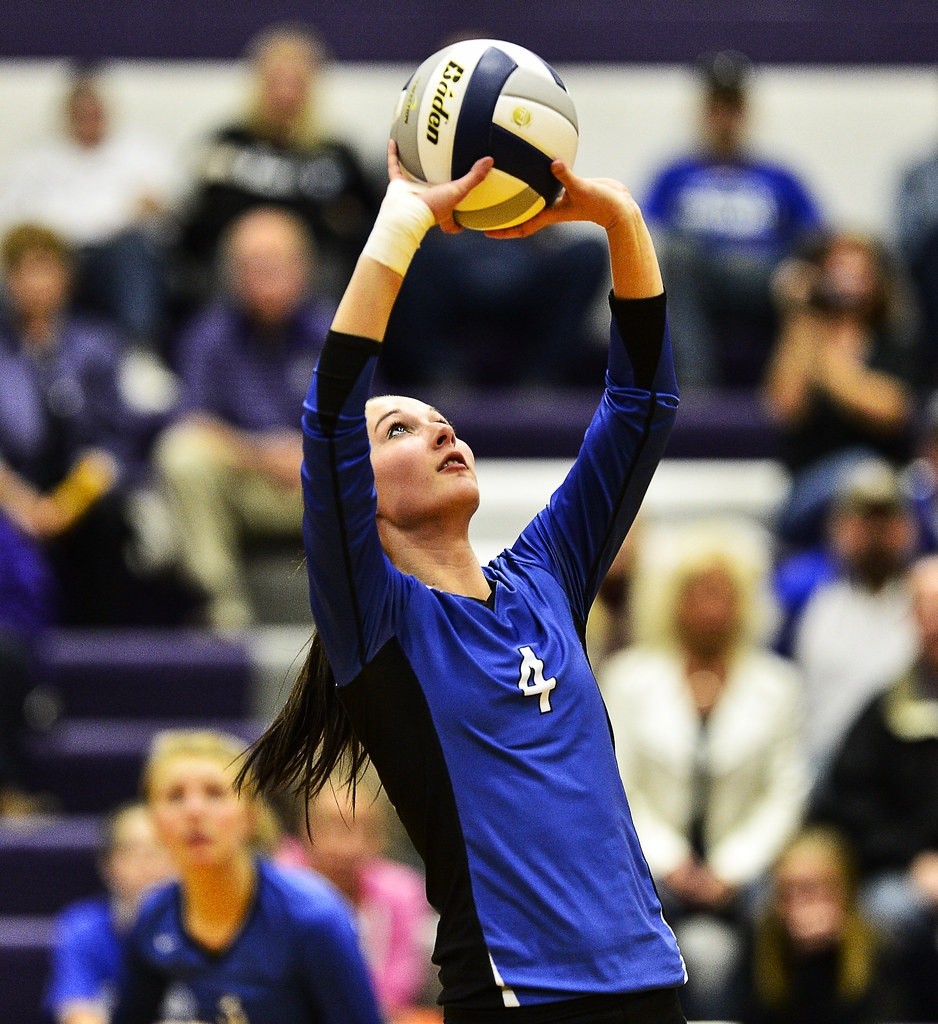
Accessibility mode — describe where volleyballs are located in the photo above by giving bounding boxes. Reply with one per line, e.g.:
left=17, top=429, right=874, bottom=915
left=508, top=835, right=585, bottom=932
left=385, top=36, right=583, bottom=235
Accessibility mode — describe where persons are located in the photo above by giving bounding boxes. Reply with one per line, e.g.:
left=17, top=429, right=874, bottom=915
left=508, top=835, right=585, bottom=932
left=299, top=134, right=690, bottom=1024
left=102, top=725, right=385, bottom=1024
left=1, top=22, right=937, bottom=1024
